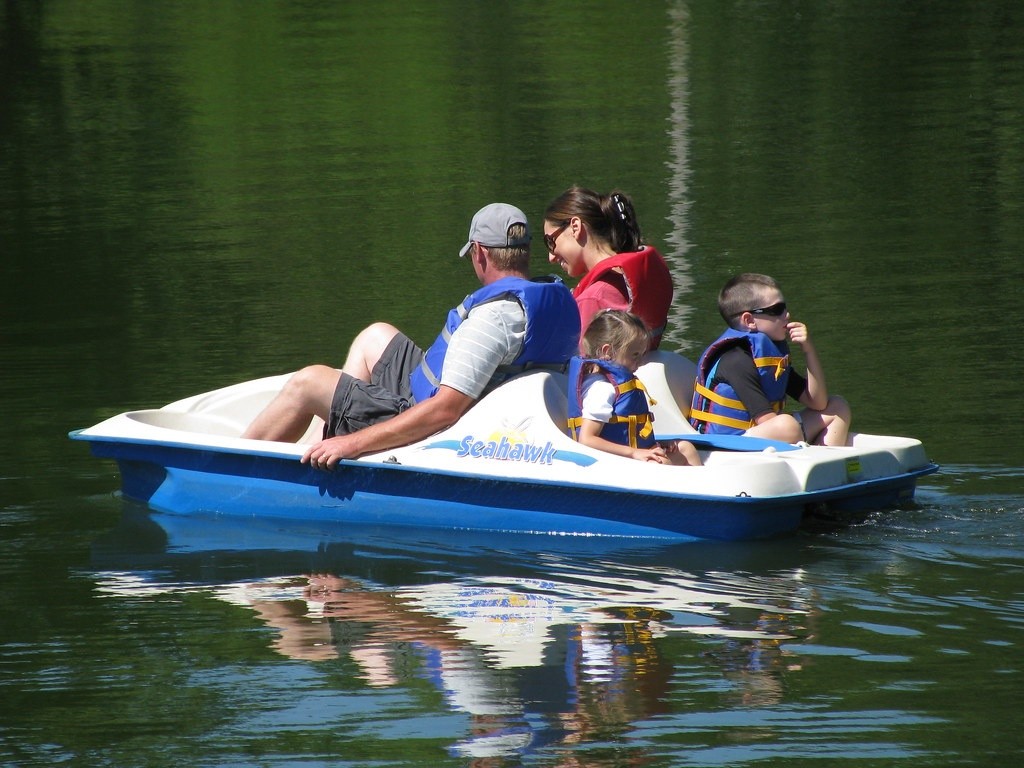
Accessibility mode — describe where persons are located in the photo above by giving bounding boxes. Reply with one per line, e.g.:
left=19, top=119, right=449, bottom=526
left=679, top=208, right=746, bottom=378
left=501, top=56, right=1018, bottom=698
left=566, top=308, right=702, bottom=466
left=240, top=202, right=581, bottom=471
left=688, top=273, right=851, bottom=447
left=542, top=186, right=674, bottom=356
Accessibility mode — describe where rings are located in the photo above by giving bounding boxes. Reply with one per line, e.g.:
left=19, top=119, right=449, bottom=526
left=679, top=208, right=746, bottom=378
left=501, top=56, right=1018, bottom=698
left=323, top=454, right=328, bottom=460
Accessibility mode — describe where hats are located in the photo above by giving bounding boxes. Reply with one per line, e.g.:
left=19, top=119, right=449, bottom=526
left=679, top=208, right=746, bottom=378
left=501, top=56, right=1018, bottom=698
left=458, top=202, right=531, bottom=257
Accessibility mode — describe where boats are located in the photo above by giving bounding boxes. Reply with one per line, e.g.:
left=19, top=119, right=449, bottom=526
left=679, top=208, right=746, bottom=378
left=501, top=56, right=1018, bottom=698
left=69, top=368, right=942, bottom=545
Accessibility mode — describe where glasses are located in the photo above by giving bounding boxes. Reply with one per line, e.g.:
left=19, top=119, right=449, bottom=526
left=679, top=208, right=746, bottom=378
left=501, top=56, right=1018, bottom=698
left=728, top=302, right=787, bottom=319
left=544, top=219, right=571, bottom=254
